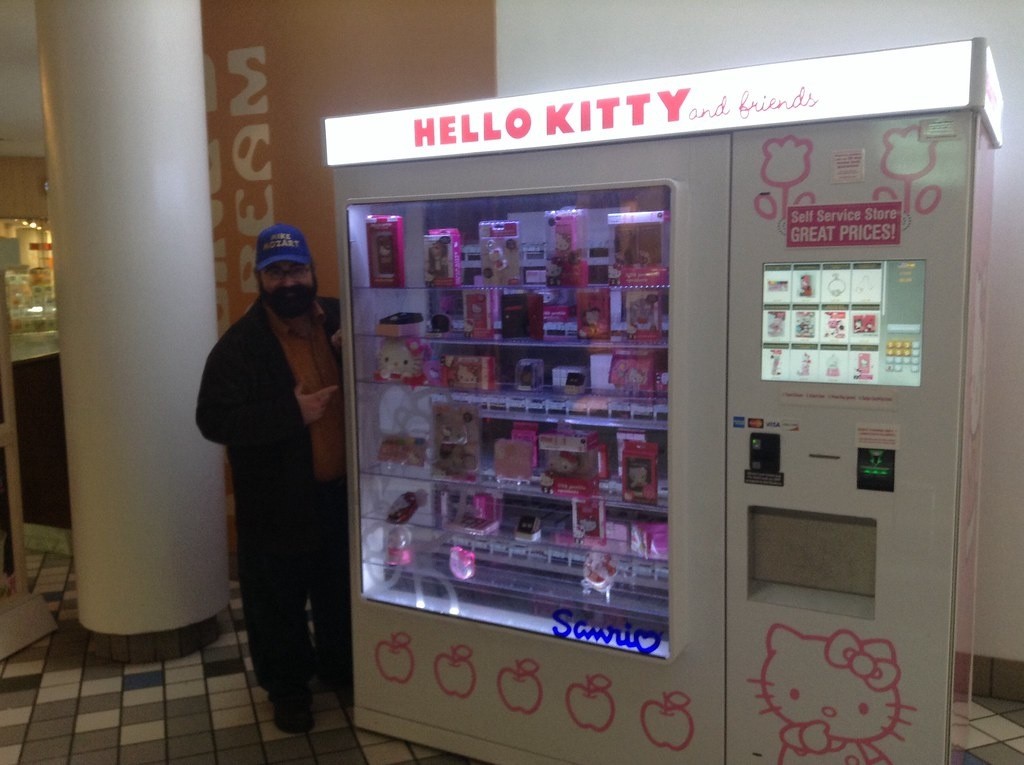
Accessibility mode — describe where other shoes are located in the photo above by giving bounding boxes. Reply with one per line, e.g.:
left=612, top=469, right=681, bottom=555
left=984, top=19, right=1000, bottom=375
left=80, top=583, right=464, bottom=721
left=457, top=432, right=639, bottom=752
left=273, top=688, right=315, bottom=734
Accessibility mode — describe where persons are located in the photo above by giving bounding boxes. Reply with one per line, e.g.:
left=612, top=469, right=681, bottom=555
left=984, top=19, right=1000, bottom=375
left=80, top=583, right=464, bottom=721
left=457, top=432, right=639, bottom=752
left=196, top=224, right=353, bottom=734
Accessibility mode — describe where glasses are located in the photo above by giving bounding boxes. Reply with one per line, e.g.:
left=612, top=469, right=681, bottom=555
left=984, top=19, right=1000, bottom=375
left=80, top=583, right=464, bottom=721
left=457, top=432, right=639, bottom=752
left=262, top=264, right=310, bottom=279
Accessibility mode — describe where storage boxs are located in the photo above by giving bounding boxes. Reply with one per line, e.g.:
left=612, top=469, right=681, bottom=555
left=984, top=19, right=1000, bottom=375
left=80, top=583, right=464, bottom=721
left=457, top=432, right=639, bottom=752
left=479, top=220, right=524, bottom=286
left=440, top=351, right=497, bottom=392
left=537, top=429, right=609, bottom=496
left=461, top=286, right=622, bottom=342
left=624, top=287, right=664, bottom=344
left=570, top=495, right=667, bottom=557
left=513, top=517, right=543, bottom=541
left=617, top=429, right=659, bottom=505
left=609, top=348, right=654, bottom=401
left=367, top=210, right=404, bottom=288
left=423, top=228, right=461, bottom=287
left=434, top=489, right=504, bottom=534
left=374, top=311, right=426, bottom=337
left=545, top=208, right=589, bottom=287
left=608, top=209, right=671, bottom=287
left=425, top=312, right=455, bottom=339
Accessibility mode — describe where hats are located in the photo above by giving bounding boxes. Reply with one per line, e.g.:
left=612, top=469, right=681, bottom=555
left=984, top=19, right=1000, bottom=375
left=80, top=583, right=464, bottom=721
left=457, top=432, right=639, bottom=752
left=256, top=223, right=311, bottom=272
